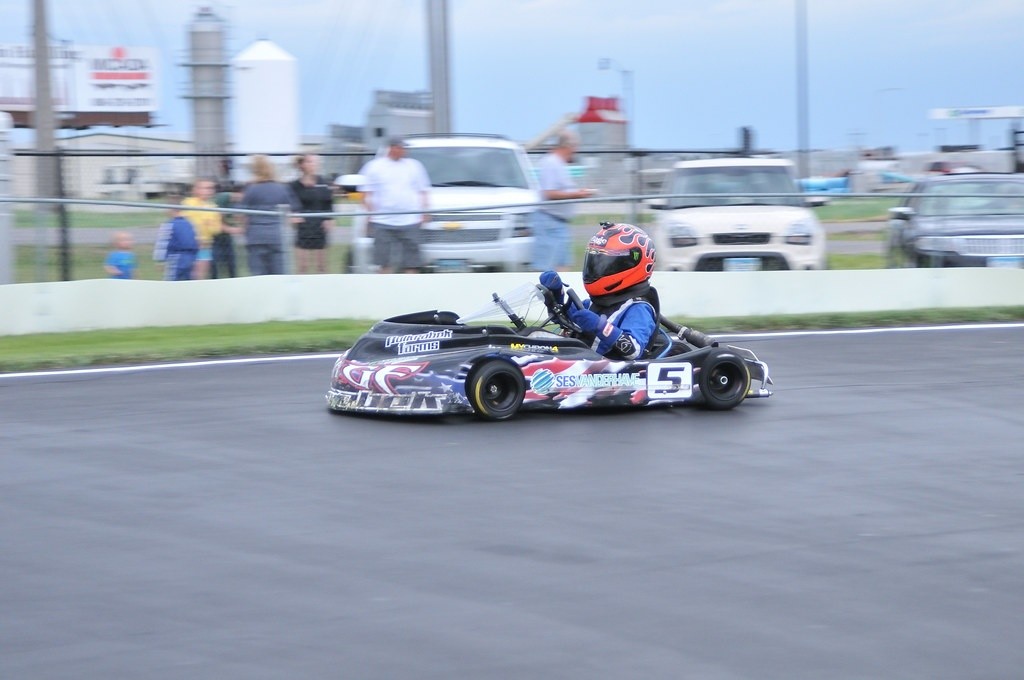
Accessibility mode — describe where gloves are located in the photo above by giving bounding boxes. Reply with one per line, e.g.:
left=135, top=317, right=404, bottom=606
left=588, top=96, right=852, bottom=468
left=572, top=309, right=623, bottom=348
left=539, top=271, right=564, bottom=312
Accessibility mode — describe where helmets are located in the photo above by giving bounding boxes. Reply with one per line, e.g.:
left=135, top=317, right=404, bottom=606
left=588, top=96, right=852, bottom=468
left=582, top=223, right=656, bottom=306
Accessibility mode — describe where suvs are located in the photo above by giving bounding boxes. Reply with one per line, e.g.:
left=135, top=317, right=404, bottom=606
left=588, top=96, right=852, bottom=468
left=336, top=129, right=542, bottom=273
left=645, top=157, right=831, bottom=273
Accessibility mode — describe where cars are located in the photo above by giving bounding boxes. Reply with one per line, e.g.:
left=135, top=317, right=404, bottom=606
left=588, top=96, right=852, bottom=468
left=885, top=171, right=1024, bottom=267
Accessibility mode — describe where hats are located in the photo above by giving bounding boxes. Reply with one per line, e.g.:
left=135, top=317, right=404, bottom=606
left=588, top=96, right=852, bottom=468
left=385, top=136, right=411, bottom=146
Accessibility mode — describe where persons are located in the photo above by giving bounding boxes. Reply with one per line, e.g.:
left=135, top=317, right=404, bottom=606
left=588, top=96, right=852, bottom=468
left=528, top=127, right=598, bottom=271
left=538, top=222, right=659, bottom=360
left=102, top=231, right=141, bottom=280
left=355, top=136, right=434, bottom=274
left=151, top=149, right=338, bottom=282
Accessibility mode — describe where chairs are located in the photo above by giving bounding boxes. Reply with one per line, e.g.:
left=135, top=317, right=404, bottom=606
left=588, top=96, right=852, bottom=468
left=642, top=286, right=661, bottom=358
